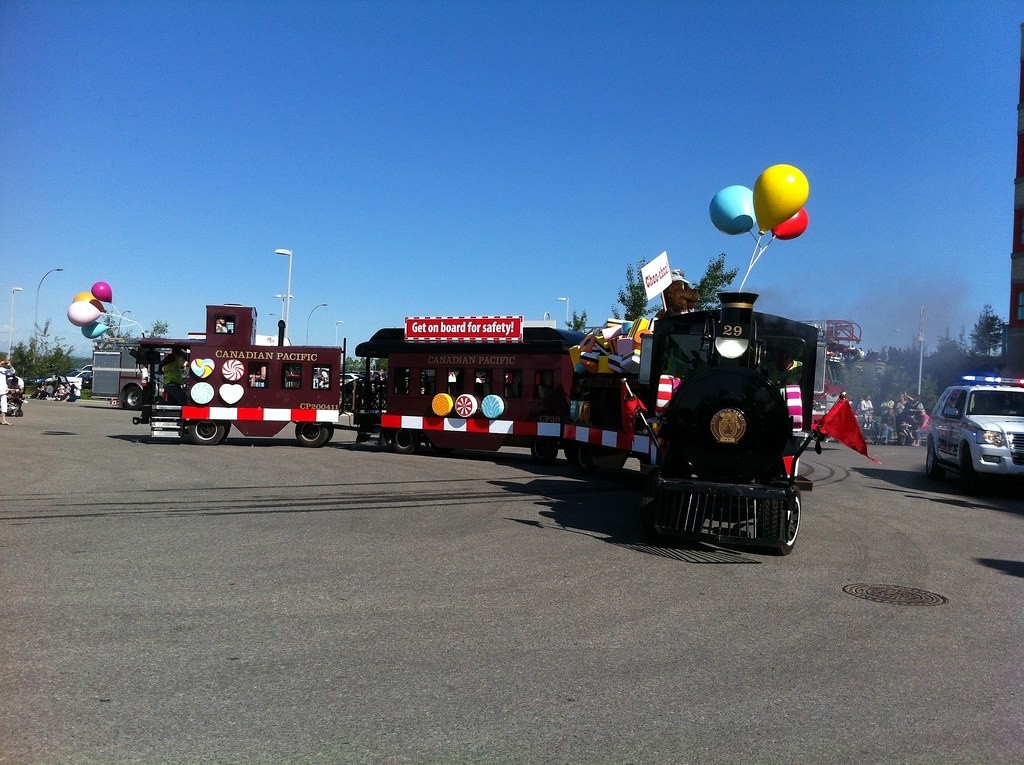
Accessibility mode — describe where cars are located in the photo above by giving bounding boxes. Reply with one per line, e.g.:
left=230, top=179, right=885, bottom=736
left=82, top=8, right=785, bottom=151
left=46, top=369, right=92, bottom=390
left=33, top=376, right=48, bottom=387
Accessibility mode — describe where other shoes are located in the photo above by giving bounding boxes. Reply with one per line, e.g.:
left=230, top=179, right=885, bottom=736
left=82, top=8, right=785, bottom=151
left=46, top=397, right=66, bottom=402
left=911, top=443, right=919, bottom=447
left=1, top=421, right=13, bottom=425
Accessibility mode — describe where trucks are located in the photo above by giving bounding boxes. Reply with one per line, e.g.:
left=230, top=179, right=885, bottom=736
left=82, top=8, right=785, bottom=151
left=92, top=334, right=291, bottom=408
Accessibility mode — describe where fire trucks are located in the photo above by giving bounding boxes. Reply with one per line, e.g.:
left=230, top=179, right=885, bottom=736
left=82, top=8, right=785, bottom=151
left=799, top=320, right=861, bottom=439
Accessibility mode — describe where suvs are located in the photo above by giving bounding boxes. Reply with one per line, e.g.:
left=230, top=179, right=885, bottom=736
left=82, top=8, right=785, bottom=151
left=925, top=375, right=1024, bottom=490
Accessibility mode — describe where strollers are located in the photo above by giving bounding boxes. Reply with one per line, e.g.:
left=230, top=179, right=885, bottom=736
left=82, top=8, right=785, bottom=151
left=858, top=411, right=881, bottom=444
left=6, top=392, right=24, bottom=417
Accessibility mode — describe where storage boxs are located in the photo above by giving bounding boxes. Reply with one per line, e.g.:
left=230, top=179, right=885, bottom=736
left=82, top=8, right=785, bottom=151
left=568, top=317, right=654, bottom=374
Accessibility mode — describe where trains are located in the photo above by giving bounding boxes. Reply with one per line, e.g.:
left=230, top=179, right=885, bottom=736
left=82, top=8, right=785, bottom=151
left=130, top=292, right=819, bottom=557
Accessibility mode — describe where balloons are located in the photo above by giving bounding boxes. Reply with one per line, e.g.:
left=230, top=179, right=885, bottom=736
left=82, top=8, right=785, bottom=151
left=709, top=164, right=811, bottom=239
left=67, top=281, right=112, bottom=338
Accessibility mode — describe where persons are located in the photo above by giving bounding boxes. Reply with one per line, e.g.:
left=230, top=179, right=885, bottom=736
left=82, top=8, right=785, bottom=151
left=140, top=364, right=150, bottom=384
left=319, top=371, right=329, bottom=388
left=0, top=361, right=24, bottom=425
left=849, top=391, right=930, bottom=447
left=447, top=371, right=457, bottom=383
left=250, top=366, right=267, bottom=382
left=354, top=369, right=386, bottom=413
left=215, top=316, right=232, bottom=333
left=27, top=377, right=81, bottom=402
left=476, top=375, right=486, bottom=384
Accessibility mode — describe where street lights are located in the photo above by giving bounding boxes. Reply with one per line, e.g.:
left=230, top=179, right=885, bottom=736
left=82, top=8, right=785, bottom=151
left=117, top=311, right=132, bottom=338
left=34, top=269, right=64, bottom=365
left=7, top=286, right=25, bottom=363
left=274, top=291, right=293, bottom=337
left=915, top=330, right=927, bottom=395
left=274, top=248, right=294, bottom=343
left=335, top=320, right=345, bottom=348
left=304, top=304, right=328, bottom=347
left=555, top=296, right=570, bottom=330
